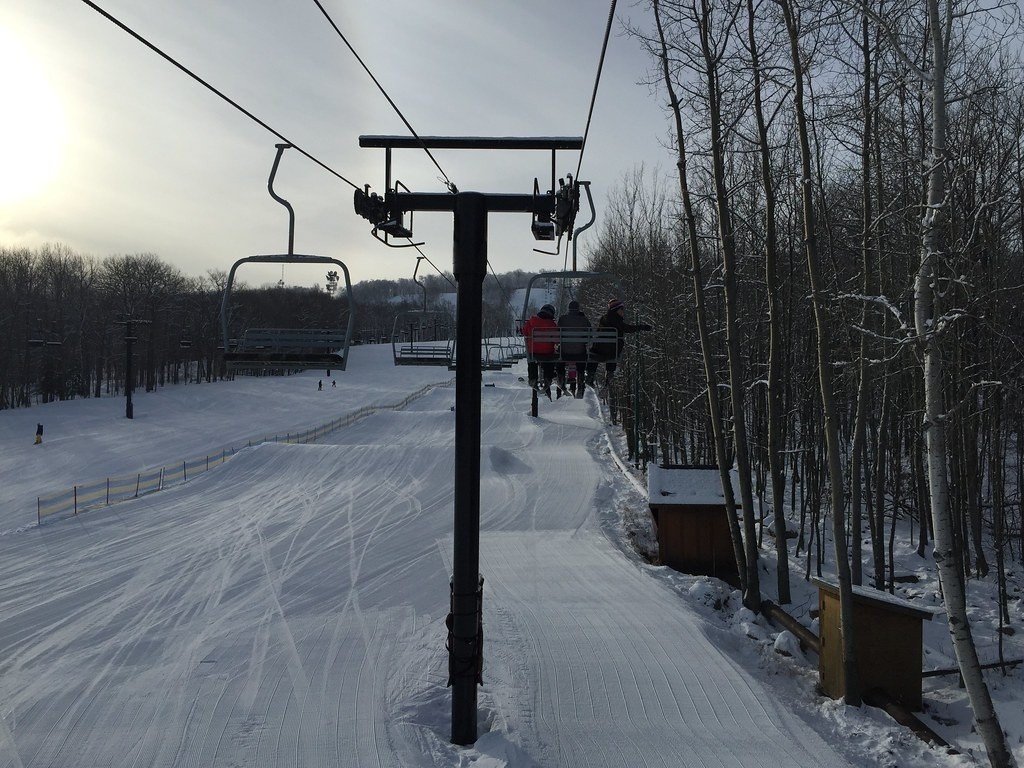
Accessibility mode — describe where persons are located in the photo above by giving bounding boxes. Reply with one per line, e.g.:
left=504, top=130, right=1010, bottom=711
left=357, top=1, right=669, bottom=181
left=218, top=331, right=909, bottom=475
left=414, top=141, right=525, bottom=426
left=585, top=299, right=654, bottom=381
left=318, top=380, right=322, bottom=391
left=555, top=364, right=576, bottom=400
left=557, top=300, right=592, bottom=399
left=331, top=380, right=337, bottom=387
left=522, top=304, right=560, bottom=392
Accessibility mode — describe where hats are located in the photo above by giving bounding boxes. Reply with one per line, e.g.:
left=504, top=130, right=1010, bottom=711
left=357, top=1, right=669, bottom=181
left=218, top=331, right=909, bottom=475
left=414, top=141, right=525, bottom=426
left=539, top=304, right=556, bottom=317
left=568, top=301, right=580, bottom=310
left=606, top=299, right=626, bottom=309
